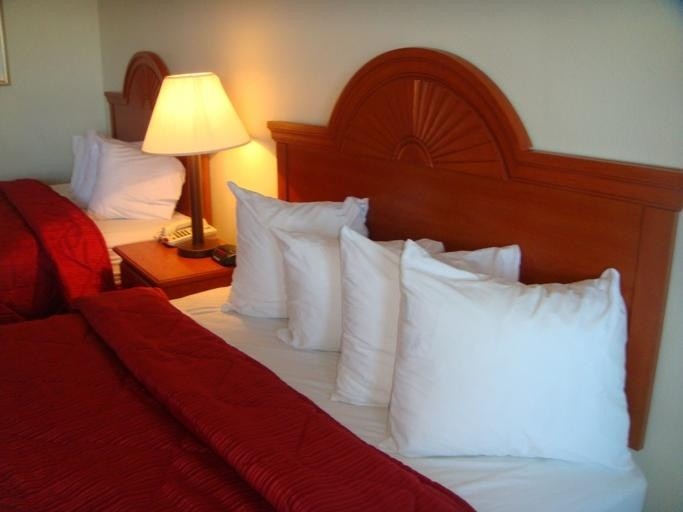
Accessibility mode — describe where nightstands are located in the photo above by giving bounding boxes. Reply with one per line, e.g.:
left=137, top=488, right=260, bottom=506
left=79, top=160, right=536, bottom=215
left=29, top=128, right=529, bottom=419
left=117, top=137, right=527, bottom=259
left=112, top=237, right=236, bottom=301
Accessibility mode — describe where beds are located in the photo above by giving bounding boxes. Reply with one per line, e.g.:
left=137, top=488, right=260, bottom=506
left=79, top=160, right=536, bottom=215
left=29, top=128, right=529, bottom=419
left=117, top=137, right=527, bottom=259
left=0, top=48, right=683, bottom=511
left=0, top=51, right=212, bottom=324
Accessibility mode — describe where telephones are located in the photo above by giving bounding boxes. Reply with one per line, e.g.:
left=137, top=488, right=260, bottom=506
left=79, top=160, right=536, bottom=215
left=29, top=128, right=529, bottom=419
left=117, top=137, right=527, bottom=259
left=154, top=217, right=217, bottom=247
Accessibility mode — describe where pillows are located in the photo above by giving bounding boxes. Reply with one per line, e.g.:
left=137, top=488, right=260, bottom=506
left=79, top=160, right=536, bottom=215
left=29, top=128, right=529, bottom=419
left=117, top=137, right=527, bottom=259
left=270, top=228, right=443, bottom=353
left=224, top=183, right=369, bottom=320
left=331, top=226, right=521, bottom=408
left=67, top=128, right=185, bottom=221
left=386, top=239, right=633, bottom=472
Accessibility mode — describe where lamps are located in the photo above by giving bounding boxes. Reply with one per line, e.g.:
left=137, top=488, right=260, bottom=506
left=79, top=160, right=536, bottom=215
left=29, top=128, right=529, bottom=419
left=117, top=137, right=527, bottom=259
left=142, top=72, right=251, bottom=257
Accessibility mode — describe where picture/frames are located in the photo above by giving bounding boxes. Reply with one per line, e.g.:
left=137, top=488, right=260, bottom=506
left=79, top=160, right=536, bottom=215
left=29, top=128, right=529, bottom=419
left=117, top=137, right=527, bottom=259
left=0, top=0, right=11, bottom=86
